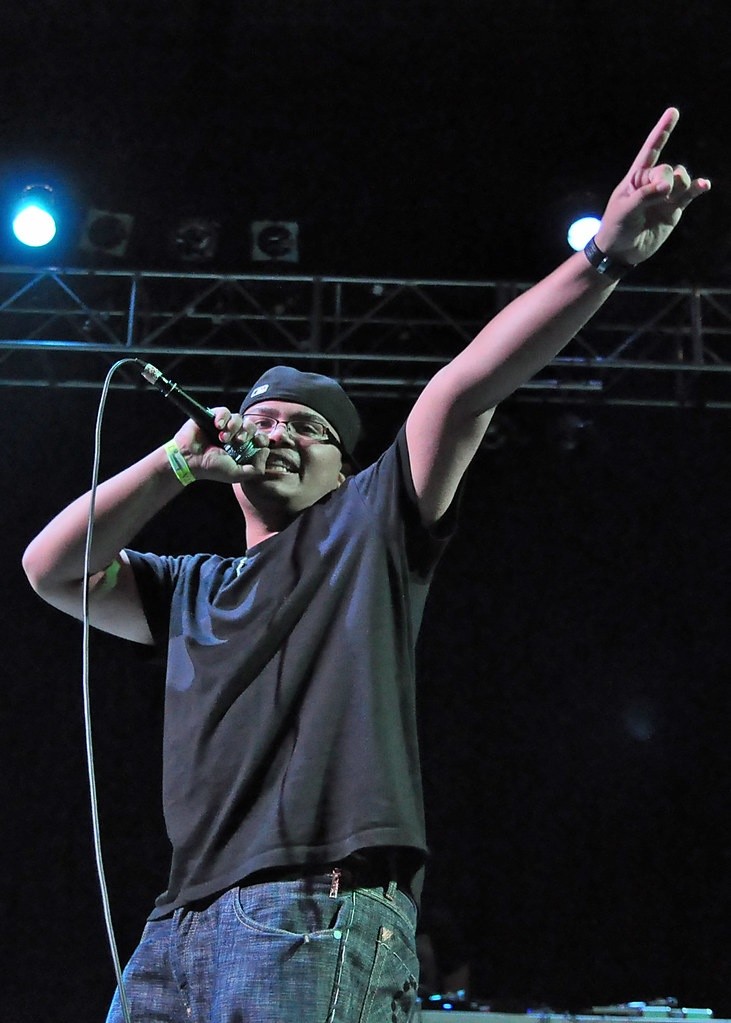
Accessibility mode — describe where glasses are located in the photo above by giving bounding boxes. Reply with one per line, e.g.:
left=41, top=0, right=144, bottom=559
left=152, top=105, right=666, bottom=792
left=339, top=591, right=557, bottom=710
left=242, top=414, right=348, bottom=463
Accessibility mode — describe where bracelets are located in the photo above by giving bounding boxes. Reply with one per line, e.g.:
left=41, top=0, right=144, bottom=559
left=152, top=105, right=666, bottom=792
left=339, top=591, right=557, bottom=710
left=164, top=440, right=196, bottom=488
left=585, top=237, right=633, bottom=281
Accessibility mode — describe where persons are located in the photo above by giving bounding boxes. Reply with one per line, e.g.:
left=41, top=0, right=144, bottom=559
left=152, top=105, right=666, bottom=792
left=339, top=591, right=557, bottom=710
left=21, top=105, right=713, bottom=1021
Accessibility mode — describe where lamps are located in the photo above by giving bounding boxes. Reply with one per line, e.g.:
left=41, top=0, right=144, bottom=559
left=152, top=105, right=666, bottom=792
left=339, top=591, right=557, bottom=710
left=8, top=184, right=61, bottom=263
left=560, top=190, right=603, bottom=252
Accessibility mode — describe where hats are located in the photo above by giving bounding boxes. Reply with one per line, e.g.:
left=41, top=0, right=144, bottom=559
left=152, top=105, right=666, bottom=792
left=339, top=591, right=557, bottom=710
left=239, top=366, right=364, bottom=474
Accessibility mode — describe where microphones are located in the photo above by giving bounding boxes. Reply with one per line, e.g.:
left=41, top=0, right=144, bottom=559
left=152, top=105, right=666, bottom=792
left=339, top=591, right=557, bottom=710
left=134, top=359, right=256, bottom=466
